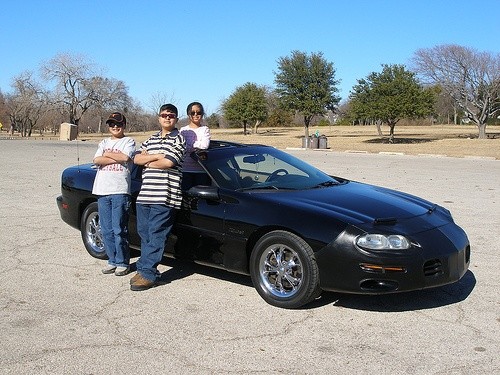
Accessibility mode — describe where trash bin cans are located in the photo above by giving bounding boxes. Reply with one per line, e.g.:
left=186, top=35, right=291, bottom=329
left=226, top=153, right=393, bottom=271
left=319, top=134, right=327, bottom=149
left=309, top=134, right=318, bottom=149
left=301, top=136, right=311, bottom=148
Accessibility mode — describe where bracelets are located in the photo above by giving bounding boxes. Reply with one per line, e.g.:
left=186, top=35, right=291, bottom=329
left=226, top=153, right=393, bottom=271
left=147, top=162, right=150, bottom=168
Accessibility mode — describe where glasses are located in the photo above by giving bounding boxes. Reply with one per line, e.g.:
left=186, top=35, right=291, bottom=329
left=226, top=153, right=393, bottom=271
left=108, top=122, right=124, bottom=127
left=188, top=112, right=203, bottom=115
left=159, top=114, right=177, bottom=118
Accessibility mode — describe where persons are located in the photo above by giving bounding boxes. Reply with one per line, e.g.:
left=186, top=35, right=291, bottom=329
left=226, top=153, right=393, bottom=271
left=91, top=113, right=136, bottom=276
left=178, top=102, right=211, bottom=185
left=130, top=103, right=186, bottom=291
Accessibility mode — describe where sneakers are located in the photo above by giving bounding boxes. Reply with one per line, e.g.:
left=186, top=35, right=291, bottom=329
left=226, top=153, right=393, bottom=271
left=102, top=263, right=116, bottom=274
left=131, top=276, right=160, bottom=291
left=130, top=273, right=142, bottom=285
left=115, top=266, right=131, bottom=276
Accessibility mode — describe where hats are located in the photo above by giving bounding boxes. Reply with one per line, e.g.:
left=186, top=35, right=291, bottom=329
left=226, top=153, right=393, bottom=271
left=106, top=112, right=126, bottom=125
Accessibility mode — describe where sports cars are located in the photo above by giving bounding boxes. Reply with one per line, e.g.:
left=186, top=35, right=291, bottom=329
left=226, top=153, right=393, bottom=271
left=56, top=140, right=470, bottom=308
